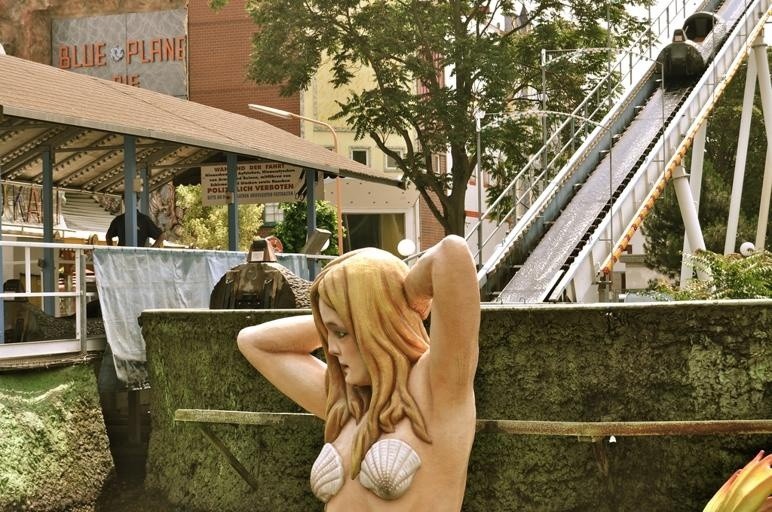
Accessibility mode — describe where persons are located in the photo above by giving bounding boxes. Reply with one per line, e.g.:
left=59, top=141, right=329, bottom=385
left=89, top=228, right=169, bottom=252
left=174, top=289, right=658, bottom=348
left=106, top=191, right=165, bottom=247
left=237, top=234, right=481, bottom=511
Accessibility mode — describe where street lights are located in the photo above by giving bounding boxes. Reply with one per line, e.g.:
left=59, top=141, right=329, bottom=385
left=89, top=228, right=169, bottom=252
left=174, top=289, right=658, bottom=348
left=247, top=102, right=345, bottom=260
